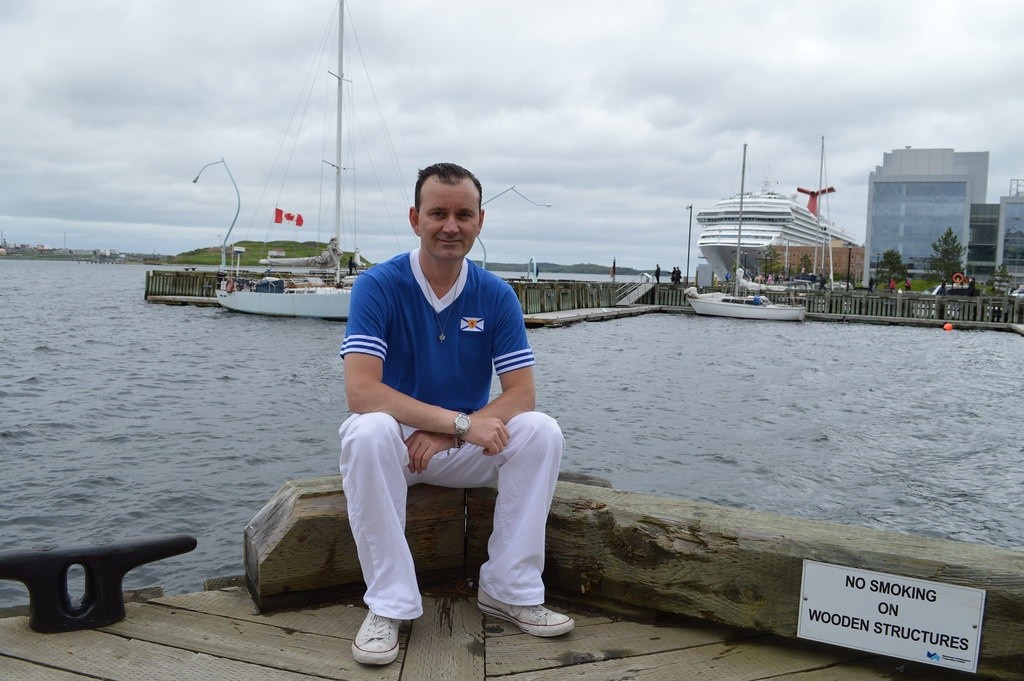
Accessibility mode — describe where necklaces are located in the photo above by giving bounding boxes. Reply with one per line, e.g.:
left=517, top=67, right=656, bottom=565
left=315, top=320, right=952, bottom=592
left=419, top=259, right=462, bottom=343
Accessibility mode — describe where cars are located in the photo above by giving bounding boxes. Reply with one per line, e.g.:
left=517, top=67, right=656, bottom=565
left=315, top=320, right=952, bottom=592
left=786, top=279, right=854, bottom=291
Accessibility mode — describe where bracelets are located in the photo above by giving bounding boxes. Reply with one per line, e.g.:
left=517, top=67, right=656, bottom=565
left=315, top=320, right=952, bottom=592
left=447, top=434, right=466, bottom=456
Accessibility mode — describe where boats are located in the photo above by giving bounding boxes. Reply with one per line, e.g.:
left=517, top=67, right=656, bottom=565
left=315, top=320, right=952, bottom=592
left=1012, top=287, right=1023, bottom=302
left=914, top=284, right=990, bottom=319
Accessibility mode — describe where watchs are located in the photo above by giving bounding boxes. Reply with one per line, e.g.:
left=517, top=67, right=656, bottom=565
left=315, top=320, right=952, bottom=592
left=453, top=411, right=472, bottom=439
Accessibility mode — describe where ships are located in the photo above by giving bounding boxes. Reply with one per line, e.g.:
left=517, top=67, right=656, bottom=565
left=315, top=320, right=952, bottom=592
left=696, top=179, right=858, bottom=281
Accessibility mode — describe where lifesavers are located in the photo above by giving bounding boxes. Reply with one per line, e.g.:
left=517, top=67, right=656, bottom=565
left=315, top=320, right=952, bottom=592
left=953, top=273, right=964, bottom=283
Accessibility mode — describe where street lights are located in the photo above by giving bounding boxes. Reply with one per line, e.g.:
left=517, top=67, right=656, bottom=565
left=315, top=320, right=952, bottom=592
left=193, top=157, right=241, bottom=268
left=476, top=186, right=552, bottom=269
left=846, top=242, right=852, bottom=290
left=876, top=252, right=879, bottom=287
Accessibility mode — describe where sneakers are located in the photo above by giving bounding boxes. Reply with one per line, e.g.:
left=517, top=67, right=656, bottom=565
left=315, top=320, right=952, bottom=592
left=350, top=612, right=400, bottom=665
left=477, top=588, right=576, bottom=638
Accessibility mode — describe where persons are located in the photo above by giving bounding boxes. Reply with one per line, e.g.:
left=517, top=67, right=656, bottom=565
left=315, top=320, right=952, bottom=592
left=339, top=163, right=575, bottom=666
left=818, top=273, right=827, bottom=291
left=654, top=263, right=661, bottom=284
left=786, top=261, right=794, bottom=281
left=904, top=276, right=911, bottom=291
left=801, top=264, right=806, bottom=280
left=668, top=266, right=682, bottom=285
left=867, top=274, right=874, bottom=294
left=349, top=256, right=353, bottom=275
left=889, top=278, right=895, bottom=294
left=941, top=279, right=947, bottom=297
left=765, top=273, right=784, bottom=285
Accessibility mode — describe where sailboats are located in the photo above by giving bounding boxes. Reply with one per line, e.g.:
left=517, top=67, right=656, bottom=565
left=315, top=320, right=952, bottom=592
left=687, top=143, right=807, bottom=322
left=214, top=0, right=419, bottom=320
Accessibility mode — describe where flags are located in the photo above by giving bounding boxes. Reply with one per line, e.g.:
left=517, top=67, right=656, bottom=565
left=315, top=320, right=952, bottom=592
left=274, top=207, right=304, bottom=227
